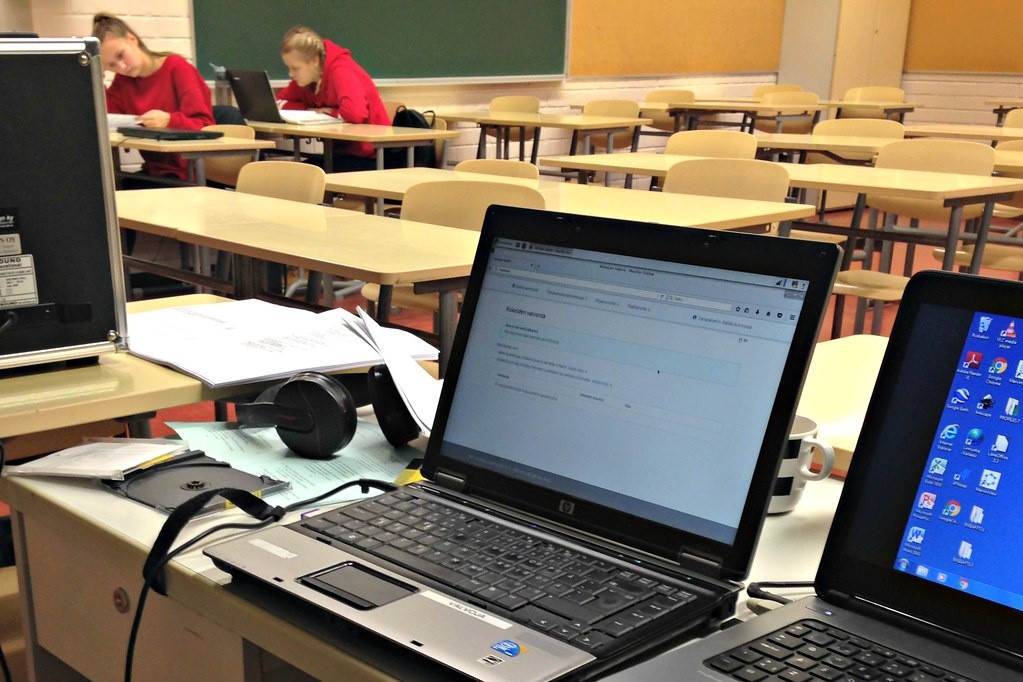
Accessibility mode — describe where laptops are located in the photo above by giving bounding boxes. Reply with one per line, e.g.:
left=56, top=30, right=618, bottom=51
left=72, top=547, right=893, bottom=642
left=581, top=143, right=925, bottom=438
left=203, top=203, right=846, bottom=682
left=225, top=68, right=286, bottom=123
left=117, top=126, right=225, bottom=141
left=594, top=270, right=1023, bottom=682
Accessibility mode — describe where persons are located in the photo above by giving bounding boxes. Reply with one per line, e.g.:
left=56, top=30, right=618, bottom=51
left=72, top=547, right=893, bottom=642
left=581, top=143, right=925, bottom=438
left=91, top=13, right=216, bottom=255
left=275, top=25, right=392, bottom=196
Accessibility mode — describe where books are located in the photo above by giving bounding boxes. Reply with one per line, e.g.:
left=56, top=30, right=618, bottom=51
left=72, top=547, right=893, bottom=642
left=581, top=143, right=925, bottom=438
left=108, top=114, right=142, bottom=133
left=808, top=414, right=865, bottom=477
left=279, top=110, right=343, bottom=124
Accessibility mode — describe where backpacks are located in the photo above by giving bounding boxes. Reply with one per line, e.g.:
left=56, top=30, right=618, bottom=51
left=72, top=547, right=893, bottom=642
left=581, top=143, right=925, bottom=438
left=384, top=105, right=437, bottom=168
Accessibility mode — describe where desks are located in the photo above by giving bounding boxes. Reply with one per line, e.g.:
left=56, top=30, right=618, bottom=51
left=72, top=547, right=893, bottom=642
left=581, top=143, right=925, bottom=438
left=0, top=99, right=1023, bottom=682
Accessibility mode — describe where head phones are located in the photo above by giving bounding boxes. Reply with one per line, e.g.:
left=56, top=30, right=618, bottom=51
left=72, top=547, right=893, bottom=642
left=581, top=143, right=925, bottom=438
left=215, top=364, right=423, bottom=460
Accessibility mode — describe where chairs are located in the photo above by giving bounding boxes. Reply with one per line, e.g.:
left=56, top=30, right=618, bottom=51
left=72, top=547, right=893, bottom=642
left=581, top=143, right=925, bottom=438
left=830, top=137, right=999, bottom=340
left=754, top=90, right=821, bottom=198
left=475, top=95, right=541, bottom=160
left=663, top=157, right=791, bottom=237
left=658, top=130, right=759, bottom=190
left=789, top=118, right=905, bottom=243
left=331, top=117, right=446, bottom=216
left=631, top=89, right=697, bottom=154
left=361, top=159, right=538, bottom=335
left=981, top=108, right=1023, bottom=219
left=399, top=181, right=546, bottom=234
left=236, top=160, right=327, bottom=205
left=195, top=123, right=253, bottom=190
left=932, top=139, right=1023, bottom=281
left=817, top=85, right=907, bottom=220
left=564, top=100, right=644, bottom=189
left=740, top=84, right=804, bottom=131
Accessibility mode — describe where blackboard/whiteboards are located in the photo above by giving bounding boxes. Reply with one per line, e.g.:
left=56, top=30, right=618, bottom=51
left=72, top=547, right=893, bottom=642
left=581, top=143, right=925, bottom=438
left=190, top=0, right=572, bottom=87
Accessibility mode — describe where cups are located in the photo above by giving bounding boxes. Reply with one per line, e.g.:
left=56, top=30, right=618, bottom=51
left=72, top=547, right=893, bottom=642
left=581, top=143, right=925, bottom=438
left=766, top=415, right=835, bottom=515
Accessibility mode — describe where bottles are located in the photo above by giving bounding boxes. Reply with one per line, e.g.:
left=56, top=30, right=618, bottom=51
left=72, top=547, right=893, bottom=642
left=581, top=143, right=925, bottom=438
left=214, top=65, right=233, bottom=107
